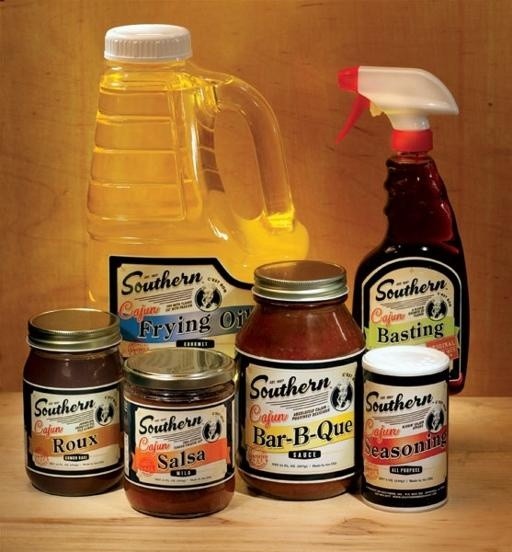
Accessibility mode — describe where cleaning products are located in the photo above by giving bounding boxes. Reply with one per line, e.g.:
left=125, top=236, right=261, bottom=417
left=332, top=64, right=470, bottom=397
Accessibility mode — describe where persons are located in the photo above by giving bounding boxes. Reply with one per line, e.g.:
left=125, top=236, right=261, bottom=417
left=429, top=410, right=443, bottom=434
left=335, top=384, right=351, bottom=410
left=205, top=421, right=219, bottom=442
left=428, top=297, right=444, bottom=320
left=198, top=286, right=219, bottom=314
left=98, top=405, right=114, bottom=425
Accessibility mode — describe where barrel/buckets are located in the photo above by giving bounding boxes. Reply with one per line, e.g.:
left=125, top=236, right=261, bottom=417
left=85, top=24, right=309, bottom=493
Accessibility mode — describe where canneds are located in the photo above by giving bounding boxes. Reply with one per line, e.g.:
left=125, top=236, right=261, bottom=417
left=22, top=307, right=125, bottom=497
left=361, top=345, right=450, bottom=513
left=233, top=259, right=368, bottom=500
left=123, top=346, right=240, bottom=518
